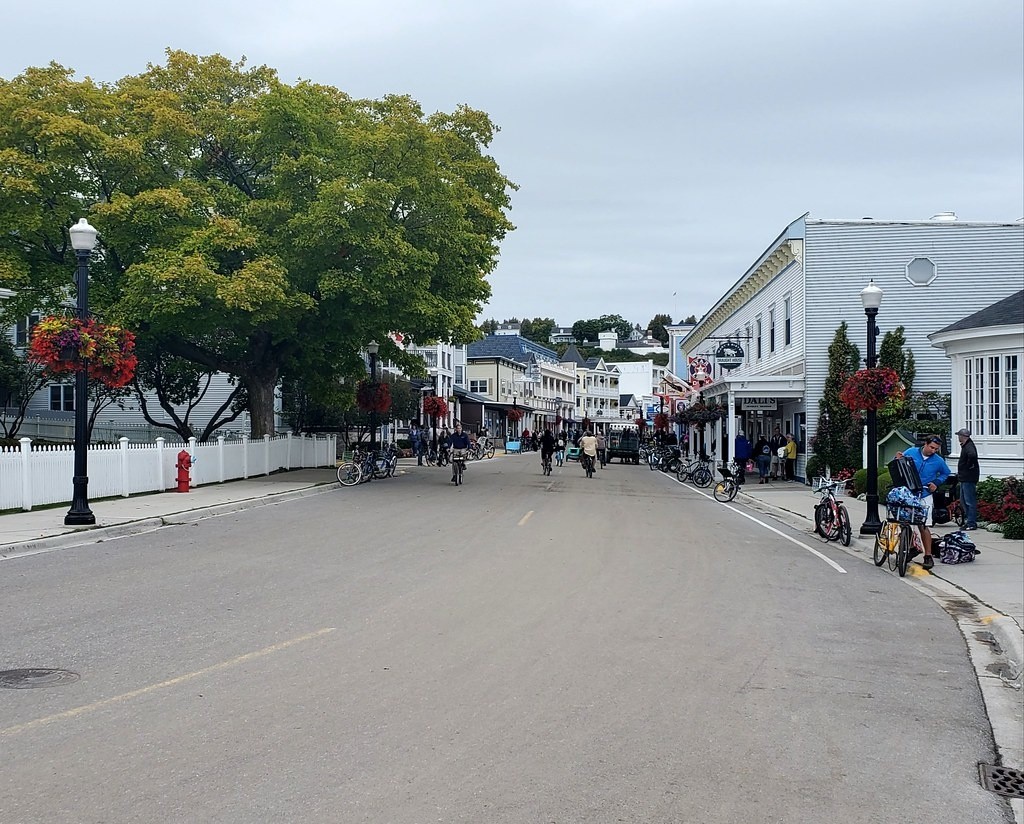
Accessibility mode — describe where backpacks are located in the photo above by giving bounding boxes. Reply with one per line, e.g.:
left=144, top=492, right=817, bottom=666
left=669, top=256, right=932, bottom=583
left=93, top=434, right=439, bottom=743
left=886, top=486, right=926, bottom=523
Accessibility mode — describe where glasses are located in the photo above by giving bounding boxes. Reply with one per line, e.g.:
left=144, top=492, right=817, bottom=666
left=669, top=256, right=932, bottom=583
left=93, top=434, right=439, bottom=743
left=932, top=438, right=942, bottom=445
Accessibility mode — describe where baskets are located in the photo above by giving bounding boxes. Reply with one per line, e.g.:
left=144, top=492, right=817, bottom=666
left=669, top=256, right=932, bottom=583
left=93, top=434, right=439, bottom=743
left=452, top=454, right=466, bottom=462
left=886, top=503, right=924, bottom=525
left=812, top=476, right=846, bottom=497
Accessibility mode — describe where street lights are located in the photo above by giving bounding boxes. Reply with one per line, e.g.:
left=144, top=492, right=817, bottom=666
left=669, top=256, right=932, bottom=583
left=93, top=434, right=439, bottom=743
left=430, top=365, right=438, bottom=454
left=512, top=390, right=518, bottom=441
left=367, top=339, right=380, bottom=464
left=638, top=401, right=643, bottom=444
left=859, top=278, right=883, bottom=535
left=62, top=216, right=100, bottom=526
left=659, top=386, right=665, bottom=446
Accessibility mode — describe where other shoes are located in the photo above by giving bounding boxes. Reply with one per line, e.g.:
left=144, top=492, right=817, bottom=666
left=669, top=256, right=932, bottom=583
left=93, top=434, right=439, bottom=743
left=738, top=475, right=786, bottom=486
left=462, top=464, right=465, bottom=470
left=959, top=524, right=977, bottom=531
left=788, top=480, right=795, bottom=482
left=583, top=465, right=586, bottom=469
left=592, top=467, right=596, bottom=473
left=923, top=555, right=934, bottom=569
left=451, top=475, right=456, bottom=481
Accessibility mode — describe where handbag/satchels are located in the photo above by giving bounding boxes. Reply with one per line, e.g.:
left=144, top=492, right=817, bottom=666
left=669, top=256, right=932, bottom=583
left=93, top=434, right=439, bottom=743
left=931, top=530, right=981, bottom=564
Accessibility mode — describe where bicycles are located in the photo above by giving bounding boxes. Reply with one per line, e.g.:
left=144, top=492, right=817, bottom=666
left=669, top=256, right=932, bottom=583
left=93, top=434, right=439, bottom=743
left=873, top=484, right=929, bottom=578
left=517, top=438, right=746, bottom=503
left=336, top=436, right=496, bottom=486
left=812, top=478, right=859, bottom=547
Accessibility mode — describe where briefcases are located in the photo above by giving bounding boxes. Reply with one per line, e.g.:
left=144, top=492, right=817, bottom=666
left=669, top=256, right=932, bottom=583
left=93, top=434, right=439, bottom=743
left=886, top=455, right=923, bottom=493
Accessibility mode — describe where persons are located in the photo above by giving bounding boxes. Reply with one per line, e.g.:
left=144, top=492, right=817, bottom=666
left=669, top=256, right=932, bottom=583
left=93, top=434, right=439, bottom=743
left=893, top=434, right=951, bottom=569
left=736, top=427, right=797, bottom=484
left=408, top=424, right=689, bottom=466
left=541, top=429, right=555, bottom=471
left=444, top=425, right=474, bottom=482
left=955, top=429, right=980, bottom=531
left=579, top=430, right=597, bottom=473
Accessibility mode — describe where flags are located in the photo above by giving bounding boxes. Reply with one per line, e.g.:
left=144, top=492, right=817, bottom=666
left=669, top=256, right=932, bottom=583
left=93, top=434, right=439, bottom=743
left=654, top=365, right=693, bottom=403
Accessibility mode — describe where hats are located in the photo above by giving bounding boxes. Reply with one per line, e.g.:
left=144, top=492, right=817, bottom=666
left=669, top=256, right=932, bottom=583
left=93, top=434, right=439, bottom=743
left=955, top=429, right=971, bottom=437
left=774, top=426, right=780, bottom=430
left=738, top=430, right=744, bottom=436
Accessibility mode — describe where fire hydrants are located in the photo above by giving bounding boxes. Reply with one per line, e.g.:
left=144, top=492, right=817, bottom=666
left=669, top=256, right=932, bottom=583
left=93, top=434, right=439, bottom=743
left=173, top=448, right=196, bottom=494
